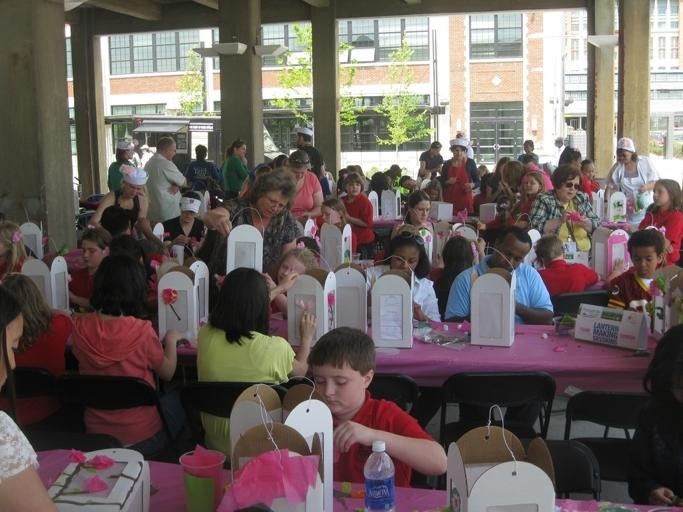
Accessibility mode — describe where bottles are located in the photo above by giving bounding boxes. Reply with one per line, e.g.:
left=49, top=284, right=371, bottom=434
left=362, top=438, right=396, bottom=512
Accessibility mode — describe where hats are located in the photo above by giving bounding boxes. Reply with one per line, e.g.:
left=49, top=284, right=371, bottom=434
left=180, top=197, right=201, bottom=214
left=298, top=127, right=314, bottom=136
left=118, top=141, right=135, bottom=150
left=119, top=164, right=149, bottom=185
left=449, top=138, right=469, bottom=147
left=616, top=137, right=634, bottom=153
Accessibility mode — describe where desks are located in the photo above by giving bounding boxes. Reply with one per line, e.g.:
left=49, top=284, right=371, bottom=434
left=170, top=312, right=663, bottom=384
left=1, top=447, right=681, bottom=511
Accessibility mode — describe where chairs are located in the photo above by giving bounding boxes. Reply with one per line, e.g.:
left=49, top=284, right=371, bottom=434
left=563, top=387, right=658, bottom=490
left=369, top=371, right=425, bottom=429
left=182, top=380, right=290, bottom=460
left=518, top=437, right=602, bottom=500
left=65, top=369, right=176, bottom=454
left=0, top=365, right=67, bottom=450
left=435, top=368, right=556, bottom=489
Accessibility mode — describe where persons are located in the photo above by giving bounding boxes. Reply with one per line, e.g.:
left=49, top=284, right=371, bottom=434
left=624, top=326, right=681, bottom=508
left=304, top=325, right=447, bottom=488
left=0, top=282, right=60, bottom=511
left=1, top=125, right=683, bottom=325
left=65, top=248, right=180, bottom=459
left=194, top=266, right=315, bottom=463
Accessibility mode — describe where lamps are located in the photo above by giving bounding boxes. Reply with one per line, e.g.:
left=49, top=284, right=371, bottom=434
left=254, top=43, right=289, bottom=57
left=191, top=47, right=219, bottom=57
left=213, top=42, right=247, bottom=55
left=586, top=35, right=618, bottom=48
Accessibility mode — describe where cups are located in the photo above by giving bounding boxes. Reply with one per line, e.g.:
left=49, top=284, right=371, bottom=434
left=552, top=316, right=575, bottom=353
left=179, top=450, right=227, bottom=512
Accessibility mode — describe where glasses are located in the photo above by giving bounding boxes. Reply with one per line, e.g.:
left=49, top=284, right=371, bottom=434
left=295, top=158, right=309, bottom=164
left=400, top=231, right=424, bottom=246
left=565, top=182, right=579, bottom=189
